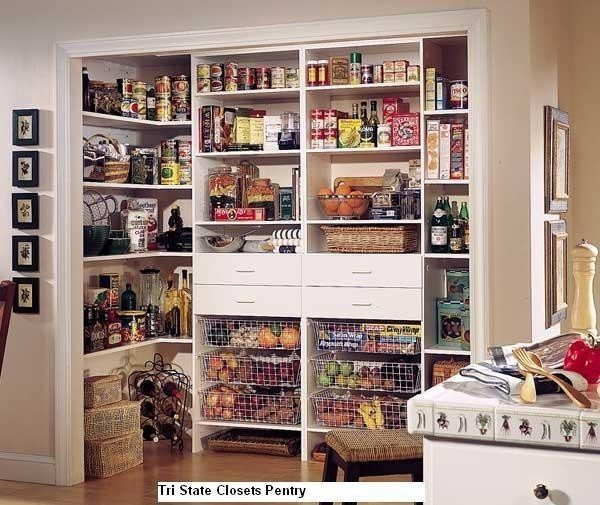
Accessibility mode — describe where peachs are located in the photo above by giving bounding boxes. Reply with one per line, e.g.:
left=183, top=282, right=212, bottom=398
left=280, top=328, right=300, bottom=348
left=257, top=327, right=279, bottom=348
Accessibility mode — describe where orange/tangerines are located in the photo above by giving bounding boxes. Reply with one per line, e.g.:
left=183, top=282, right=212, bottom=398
left=317, top=185, right=369, bottom=215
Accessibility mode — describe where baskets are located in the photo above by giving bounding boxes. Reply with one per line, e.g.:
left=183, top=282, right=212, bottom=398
left=310, top=320, right=420, bottom=429
left=84, top=132, right=130, bottom=183
left=432, top=358, right=470, bottom=390
left=197, top=317, right=301, bottom=425
left=311, top=443, right=332, bottom=462
left=83, top=375, right=144, bottom=479
left=208, top=429, right=301, bottom=457
left=319, top=225, right=418, bottom=254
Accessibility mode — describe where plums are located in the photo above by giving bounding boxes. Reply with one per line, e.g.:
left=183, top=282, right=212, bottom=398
left=234, top=391, right=270, bottom=419
left=251, top=361, right=301, bottom=385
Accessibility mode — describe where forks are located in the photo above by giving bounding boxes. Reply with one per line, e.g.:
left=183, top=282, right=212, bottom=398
left=511, top=347, right=591, bottom=409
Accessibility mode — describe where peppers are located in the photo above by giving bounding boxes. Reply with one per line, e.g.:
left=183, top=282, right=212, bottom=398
left=563, top=337, right=600, bottom=384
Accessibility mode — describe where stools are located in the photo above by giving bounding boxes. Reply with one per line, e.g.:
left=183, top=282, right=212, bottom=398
left=317, top=430, right=425, bottom=505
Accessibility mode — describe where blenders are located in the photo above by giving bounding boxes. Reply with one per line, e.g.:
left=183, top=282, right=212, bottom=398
left=277, top=112, right=300, bottom=149
left=138, top=266, right=164, bottom=337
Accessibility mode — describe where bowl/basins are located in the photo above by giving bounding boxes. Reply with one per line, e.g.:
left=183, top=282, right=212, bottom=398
left=86, top=224, right=131, bottom=256
left=203, top=236, right=243, bottom=252
left=316, top=194, right=371, bottom=219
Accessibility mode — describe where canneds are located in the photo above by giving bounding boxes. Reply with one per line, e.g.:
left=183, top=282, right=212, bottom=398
left=120, top=75, right=191, bottom=121
left=373, top=65, right=383, bottom=83
left=197, top=63, right=285, bottom=93
left=361, top=64, right=373, bottom=84
left=160, top=139, right=192, bottom=185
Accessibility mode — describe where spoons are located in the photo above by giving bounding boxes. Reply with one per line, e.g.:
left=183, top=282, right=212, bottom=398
left=513, top=351, right=543, bottom=404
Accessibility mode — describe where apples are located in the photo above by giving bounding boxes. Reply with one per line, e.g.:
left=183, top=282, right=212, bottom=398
left=319, top=361, right=361, bottom=388
left=207, top=352, right=251, bottom=383
left=203, top=386, right=250, bottom=420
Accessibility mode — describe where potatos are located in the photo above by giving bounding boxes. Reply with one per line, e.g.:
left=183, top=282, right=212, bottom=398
left=318, top=395, right=364, bottom=426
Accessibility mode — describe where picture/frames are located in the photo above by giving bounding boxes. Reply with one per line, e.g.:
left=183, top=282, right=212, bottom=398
left=542, top=103, right=571, bottom=215
left=12, top=150, right=40, bottom=187
left=12, top=277, right=39, bottom=315
left=11, top=110, right=40, bottom=147
left=11, top=192, right=39, bottom=230
left=12, top=235, right=39, bottom=272
left=543, top=220, right=570, bottom=329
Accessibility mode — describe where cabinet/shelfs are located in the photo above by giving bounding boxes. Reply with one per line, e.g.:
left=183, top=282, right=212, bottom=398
left=83, top=109, right=191, bottom=362
left=307, top=35, right=423, bottom=463
left=421, top=32, right=469, bottom=393
left=190, top=47, right=306, bottom=465
left=407, top=342, right=598, bottom=504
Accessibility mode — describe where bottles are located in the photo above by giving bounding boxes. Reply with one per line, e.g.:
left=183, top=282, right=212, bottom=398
left=377, top=123, right=392, bottom=147
left=94, top=138, right=113, bottom=153
left=83, top=302, right=108, bottom=323
left=318, top=60, right=330, bottom=85
left=83, top=64, right=94, bottom=109
left=133, top=378, right=181, bottom=444
left=165, top=268, right=192, bottom=337
left=121, top=283, right=136, bottom=312
left=307, top=60, right=318, bottom=85
left=432, top=194, right=470, bottom=253
left=351, top=101, right=380, bottom=124
